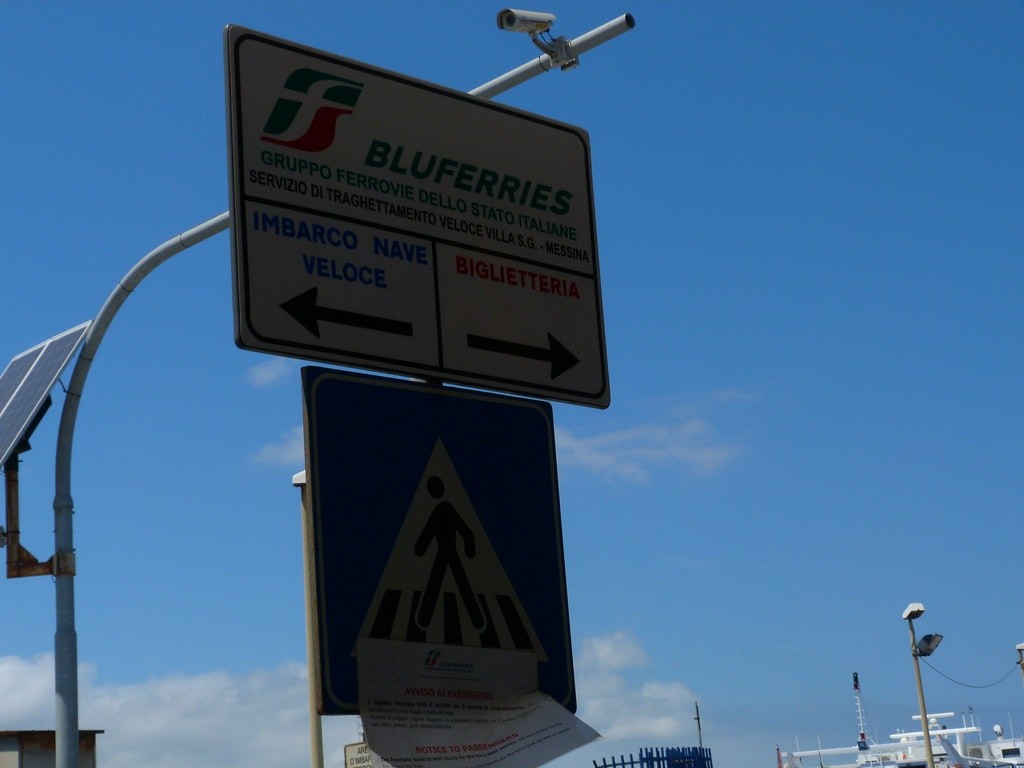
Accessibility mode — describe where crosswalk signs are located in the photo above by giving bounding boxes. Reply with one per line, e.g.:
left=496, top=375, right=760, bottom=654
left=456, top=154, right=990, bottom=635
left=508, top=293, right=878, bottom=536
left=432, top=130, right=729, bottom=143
left=302, top=365, right=578, bottom=713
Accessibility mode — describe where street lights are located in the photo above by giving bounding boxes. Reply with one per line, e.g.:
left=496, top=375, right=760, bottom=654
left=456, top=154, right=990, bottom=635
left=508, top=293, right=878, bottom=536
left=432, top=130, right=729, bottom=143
left=902, top=603, right=944, bottom=768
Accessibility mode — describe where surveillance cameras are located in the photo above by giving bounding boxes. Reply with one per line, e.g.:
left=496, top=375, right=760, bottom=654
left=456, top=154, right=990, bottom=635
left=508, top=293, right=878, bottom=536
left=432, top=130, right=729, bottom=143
left=497, top=8, right=556, bottom=35
